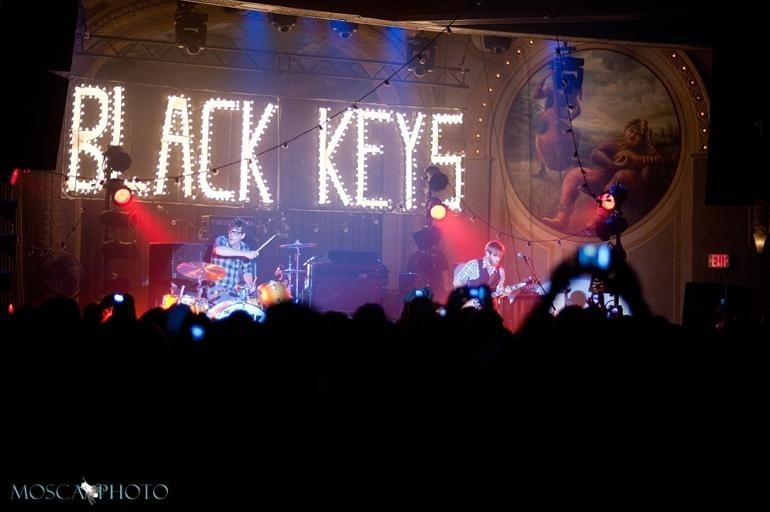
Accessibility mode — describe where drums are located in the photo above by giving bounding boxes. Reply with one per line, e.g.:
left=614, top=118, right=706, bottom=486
left=256, top=279, right=295, bottom=307
left=207, top=296, right=266, bottom=322
left=160, top=294, right=209, bottom=316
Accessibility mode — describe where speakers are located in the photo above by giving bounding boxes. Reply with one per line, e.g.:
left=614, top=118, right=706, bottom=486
left=148, top=241, right=207, bottom=311
left=703, top=124, right=760, bottom=207
left=0, top=66, right=70, bottom=170
left=681, top=280, right=751, bottom=324
left=1, top=0, right=79, bottom=72
left=309, top=278, right=382, bottom=313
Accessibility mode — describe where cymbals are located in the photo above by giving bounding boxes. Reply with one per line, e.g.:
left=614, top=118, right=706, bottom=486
left=177, top=262, right=226, bottom=280
left=275, top=244, right=318, bottom=249
left=273, top=269, right=306, bottom=274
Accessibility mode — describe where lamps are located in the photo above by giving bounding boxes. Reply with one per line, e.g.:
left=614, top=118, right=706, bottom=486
left=98, top=145, right=140, bottom=264
left=595, top=180, right=630, bottom=248
left=413, top=164, right=448, bottom=275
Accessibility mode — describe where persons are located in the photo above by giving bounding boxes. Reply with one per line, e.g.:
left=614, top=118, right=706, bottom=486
left=533, top=70, right=585, bottom=181
left=202, top=218, right=260, bottom=303
left=535, top=118, right=658, bottom=231
left=1, top=233, right=770, bottom=511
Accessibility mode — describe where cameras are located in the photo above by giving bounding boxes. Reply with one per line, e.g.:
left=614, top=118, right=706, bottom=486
left=113, top=293, right=125, bottom=306
left=188, top=324, right=203, bottom=338
left=578, top=243, right=611, bottom=270
left=414, top=289, right=422, bottom=297
left=465, top=285, right=485, bottom=304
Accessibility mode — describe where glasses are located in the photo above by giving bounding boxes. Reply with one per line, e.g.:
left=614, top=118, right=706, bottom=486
left=227, top=228, right=244, bottom=236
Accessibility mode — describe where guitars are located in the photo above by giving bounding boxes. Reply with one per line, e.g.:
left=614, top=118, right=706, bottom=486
left=458, top=276, right=539, bottom=311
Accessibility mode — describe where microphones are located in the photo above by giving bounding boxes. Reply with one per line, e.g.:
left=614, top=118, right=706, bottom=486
left=517, top=251, right=530, bottom=262
